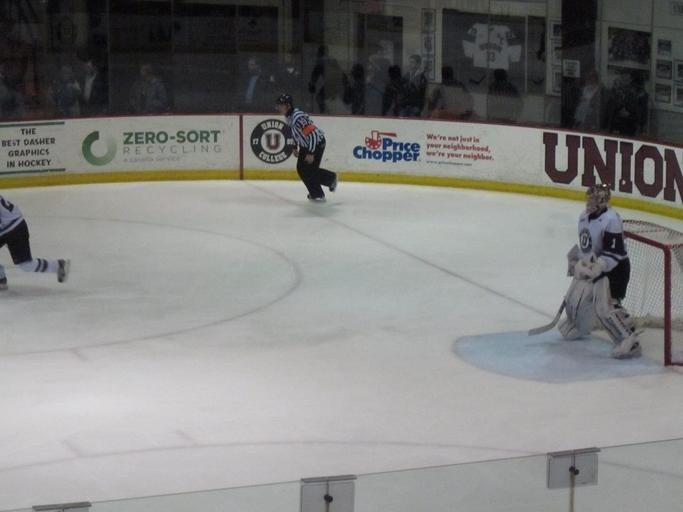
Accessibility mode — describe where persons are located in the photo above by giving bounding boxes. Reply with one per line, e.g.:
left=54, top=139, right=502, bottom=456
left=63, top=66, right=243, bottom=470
left=558, top=182, right=642, bottom=359
left=275, top=92, right=335, bottom=200
left=0, top=194, right=66, bottom=286
left=243, top=43, right=523, bottom=123
left=565, top=66, right=649, bottom=139
left=1, top=39, right=212, bottom=122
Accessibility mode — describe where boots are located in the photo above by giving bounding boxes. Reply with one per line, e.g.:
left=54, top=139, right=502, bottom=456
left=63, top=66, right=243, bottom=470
left=56, top=259, right=66, bottom=283
left=307, top=195, right=325, bottom=200
left=0, top=278, right=7, bottom=287
left=330, top=174, right=337, bottom=190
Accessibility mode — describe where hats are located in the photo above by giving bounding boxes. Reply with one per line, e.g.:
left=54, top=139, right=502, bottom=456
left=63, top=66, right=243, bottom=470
left=273, top=93, right=293, bottom=105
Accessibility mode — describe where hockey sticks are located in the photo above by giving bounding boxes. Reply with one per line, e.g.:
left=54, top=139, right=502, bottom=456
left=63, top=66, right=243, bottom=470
left=528, top=277, right=574, bottom=334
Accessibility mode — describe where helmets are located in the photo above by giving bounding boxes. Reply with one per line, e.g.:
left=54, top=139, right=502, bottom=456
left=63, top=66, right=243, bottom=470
left=586, top=184, right=611, bottom=212
